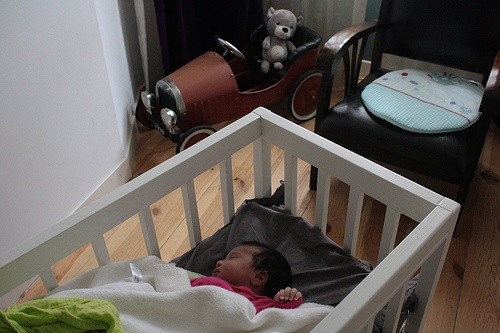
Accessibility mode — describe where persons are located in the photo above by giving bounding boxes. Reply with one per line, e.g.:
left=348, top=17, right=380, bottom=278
left=191, top=241, right=302, bottom=313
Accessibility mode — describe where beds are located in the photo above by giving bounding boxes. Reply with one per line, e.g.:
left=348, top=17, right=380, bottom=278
left=1, top=107, right=462, bottom=333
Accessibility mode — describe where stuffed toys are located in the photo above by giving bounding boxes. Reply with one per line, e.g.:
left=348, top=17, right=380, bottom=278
left=261, top=6, right=304, bottom=72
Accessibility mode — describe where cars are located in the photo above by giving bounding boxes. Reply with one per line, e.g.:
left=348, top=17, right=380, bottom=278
left=135, top=18, right=331, bottom=160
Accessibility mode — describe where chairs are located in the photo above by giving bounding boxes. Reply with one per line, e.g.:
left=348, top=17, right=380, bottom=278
left=309, top=0, right=500, bottom=235
